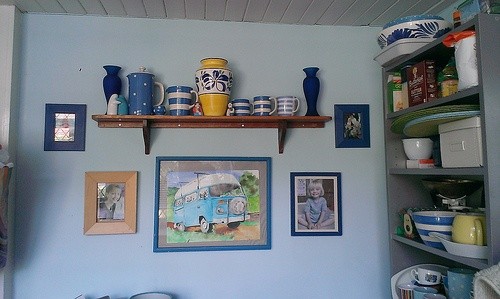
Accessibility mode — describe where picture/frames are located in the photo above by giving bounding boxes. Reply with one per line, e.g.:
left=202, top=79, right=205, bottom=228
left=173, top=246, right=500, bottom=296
left=334, top=104, right=371, bottom=149
left=152, top=156, right=272, bottom=253
left=84, top=171, right=138, bottom=235
left=43, top=104, right=86, bottom=152
left=289, top=172, right=343, bottom=236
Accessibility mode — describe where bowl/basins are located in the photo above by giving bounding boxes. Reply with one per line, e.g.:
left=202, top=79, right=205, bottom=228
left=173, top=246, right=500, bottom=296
left=377, top=15, right=451, bottom=49
left=427, top=230, right=489, bottom=261
left=402, top=137, right=435, bottom=162
left=411, top=210, right=467, bottom=250
left=390, top=262, right=449, bottom=299
left=129, top=292, right=174, bottom=299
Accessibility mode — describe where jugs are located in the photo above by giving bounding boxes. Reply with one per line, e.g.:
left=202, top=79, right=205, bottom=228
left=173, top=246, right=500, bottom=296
left=451, top=212, right=487, bottom=246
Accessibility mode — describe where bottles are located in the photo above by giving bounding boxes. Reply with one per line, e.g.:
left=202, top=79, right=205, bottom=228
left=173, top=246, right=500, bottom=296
left=101, top=65, right=122, bottom=116
left=300, top=66, right=322, bottom=117
left=195, top=58, right=234, bottom=117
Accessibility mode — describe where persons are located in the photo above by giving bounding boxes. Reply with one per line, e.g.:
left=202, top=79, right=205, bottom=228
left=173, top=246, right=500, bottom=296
left=227, top=102, right=235, bottom=116
left=299, top=181, right=334, bottom=229
left=99, top=184, right=121, bottom=219
left=345, top=115, right=361, bottom=138
left=193, top=102, right=202, bottom=116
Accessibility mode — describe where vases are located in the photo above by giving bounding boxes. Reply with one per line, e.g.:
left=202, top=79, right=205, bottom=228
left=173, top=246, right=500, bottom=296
left=302, top=67, right=321, bottom=116
left=194, top=58, right=234, bottom=117
left=103, top=64, right=122, bottom=114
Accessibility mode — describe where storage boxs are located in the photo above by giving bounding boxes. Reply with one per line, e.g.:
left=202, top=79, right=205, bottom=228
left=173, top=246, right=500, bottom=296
left=407, top=59, right=435, bottom=107
left=457, top=0, right=500, bottom=27
left=437, top=116, right=483, bottom=168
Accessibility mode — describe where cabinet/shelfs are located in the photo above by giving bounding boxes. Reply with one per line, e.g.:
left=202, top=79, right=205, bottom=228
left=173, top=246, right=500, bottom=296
left=382, top=15, right=500, bottom=279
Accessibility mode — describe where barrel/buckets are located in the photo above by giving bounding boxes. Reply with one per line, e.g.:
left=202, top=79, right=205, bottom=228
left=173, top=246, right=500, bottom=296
left=454, top=35, right=479, bottom=91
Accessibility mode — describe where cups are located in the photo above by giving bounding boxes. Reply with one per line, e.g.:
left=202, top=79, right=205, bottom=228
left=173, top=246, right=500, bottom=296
left=126, top=66, right=165, bottom=116
left=231, top=98, right=255, bottom=117
left=273, top=95, right=300, bottom=116
left=253, top=95, right=278, bottom=116
left=166, top=85, right=198, bottom=116
left=396, top=266, right=477, bottom=299
